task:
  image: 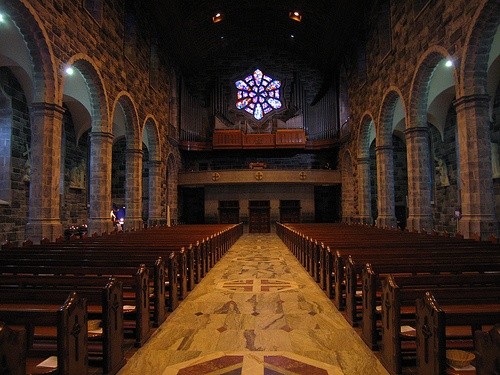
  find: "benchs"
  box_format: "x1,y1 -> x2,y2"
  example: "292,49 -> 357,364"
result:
0,220 -> 244,375
272,218 -> 499,375
